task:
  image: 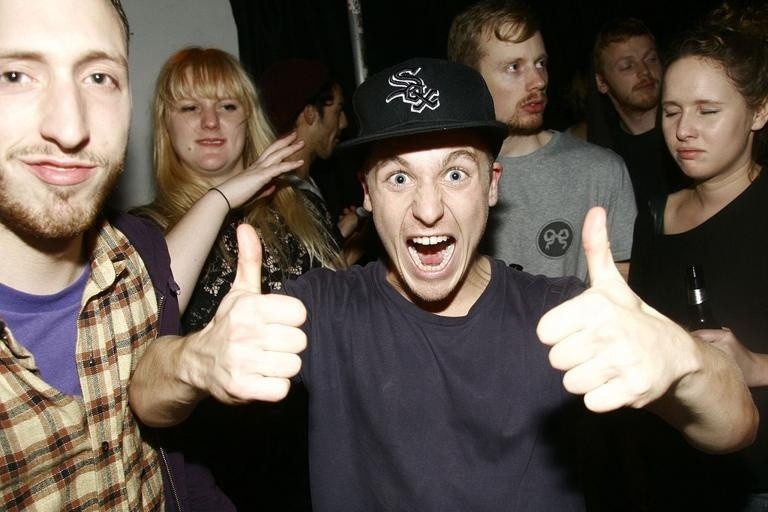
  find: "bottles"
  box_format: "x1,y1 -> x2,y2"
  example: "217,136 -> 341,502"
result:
680,266 -> 722,332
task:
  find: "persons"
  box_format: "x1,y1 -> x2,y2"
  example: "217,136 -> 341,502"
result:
126,45 -> 354,329
613,1 -> 768,510
0,0 -> 187,510
557,17 -> 692,193
255,44 -> 370,237
441,2 -> 642,297
126,46 -> 762,509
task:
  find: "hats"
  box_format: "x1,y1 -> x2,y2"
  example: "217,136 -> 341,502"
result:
334,54 -> 511,155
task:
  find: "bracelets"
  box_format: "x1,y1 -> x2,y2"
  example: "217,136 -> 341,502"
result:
205,180 -> 235,213
